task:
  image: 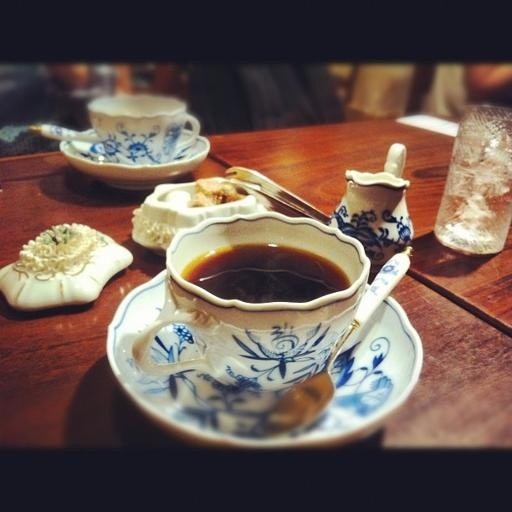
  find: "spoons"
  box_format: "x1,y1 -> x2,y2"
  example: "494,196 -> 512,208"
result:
262,247 -> 415,432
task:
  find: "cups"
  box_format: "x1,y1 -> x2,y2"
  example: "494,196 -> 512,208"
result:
88,96 -> 199,163
325,142 -> 415,279
139,216 -> 370,396
434,105 -> 511,255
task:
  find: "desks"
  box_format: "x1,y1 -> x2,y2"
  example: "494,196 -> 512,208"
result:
1,116 -> 512,449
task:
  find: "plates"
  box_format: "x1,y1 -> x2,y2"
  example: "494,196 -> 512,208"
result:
109,263 -> 424,447
60,130 -> 210,189
